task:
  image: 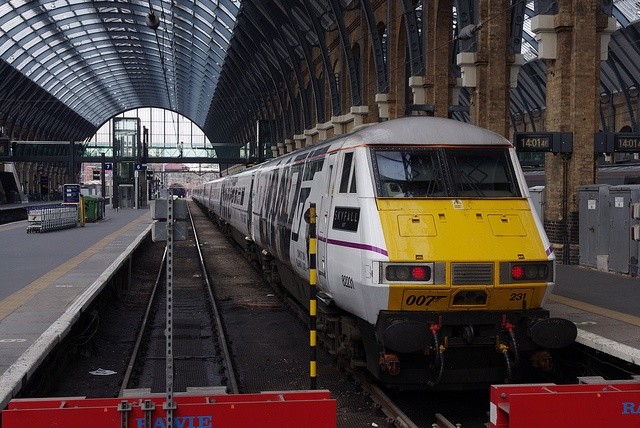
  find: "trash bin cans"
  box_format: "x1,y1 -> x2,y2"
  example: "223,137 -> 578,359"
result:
82,195 -> 98,223
96,196 -> 105,219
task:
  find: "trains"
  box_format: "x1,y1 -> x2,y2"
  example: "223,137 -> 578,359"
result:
191,116 -> 580,393
